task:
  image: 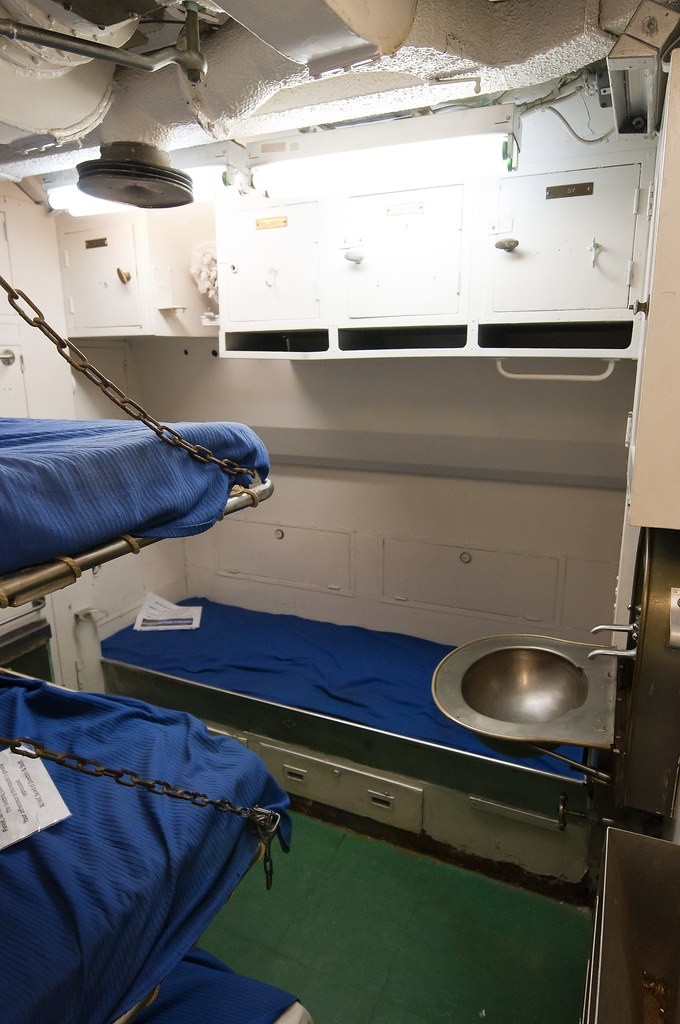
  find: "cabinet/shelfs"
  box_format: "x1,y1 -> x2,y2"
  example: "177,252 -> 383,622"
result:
214,145 -> 658,381
57,212 -> 217,339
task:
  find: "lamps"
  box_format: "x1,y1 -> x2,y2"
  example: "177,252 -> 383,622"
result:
42,139 -> 247,218
247,101 -> 522,203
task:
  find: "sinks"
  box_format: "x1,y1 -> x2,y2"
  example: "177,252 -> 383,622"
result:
431,633 -> 618,759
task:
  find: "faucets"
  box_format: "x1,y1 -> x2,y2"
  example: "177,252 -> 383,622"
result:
588,646 -> 637,661
590,616 -> 640,635
